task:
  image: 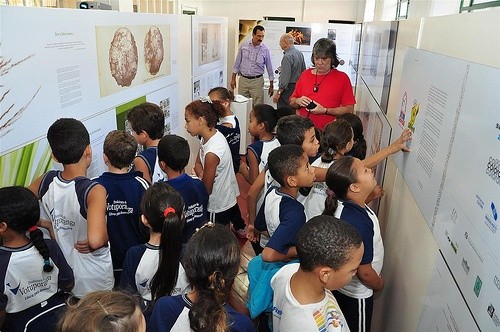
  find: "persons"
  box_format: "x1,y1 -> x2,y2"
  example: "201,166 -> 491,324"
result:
288,38 -> 356,131
273,34 -> 306,118
230,25 -> 274,108
0,87 -> 413,332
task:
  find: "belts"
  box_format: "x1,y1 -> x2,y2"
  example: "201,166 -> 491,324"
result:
241,74 -> 263,80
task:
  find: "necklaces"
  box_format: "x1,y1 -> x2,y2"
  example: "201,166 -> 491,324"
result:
313,69 -> 331,92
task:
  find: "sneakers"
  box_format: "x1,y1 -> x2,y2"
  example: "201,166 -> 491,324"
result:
232,224 -> 246,238
